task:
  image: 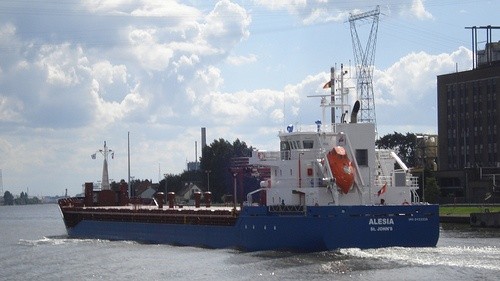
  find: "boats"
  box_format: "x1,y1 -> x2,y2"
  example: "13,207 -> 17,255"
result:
55,60 -> 441,253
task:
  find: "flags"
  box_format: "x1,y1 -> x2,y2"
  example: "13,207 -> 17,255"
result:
322,80 -> 332,89
378,184 -> 386,197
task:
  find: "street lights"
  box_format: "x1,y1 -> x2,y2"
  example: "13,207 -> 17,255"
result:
130,176 -> 135,197
232,171 -> 238,212
163,174 -> 168,204
205,169 -> 212,191
